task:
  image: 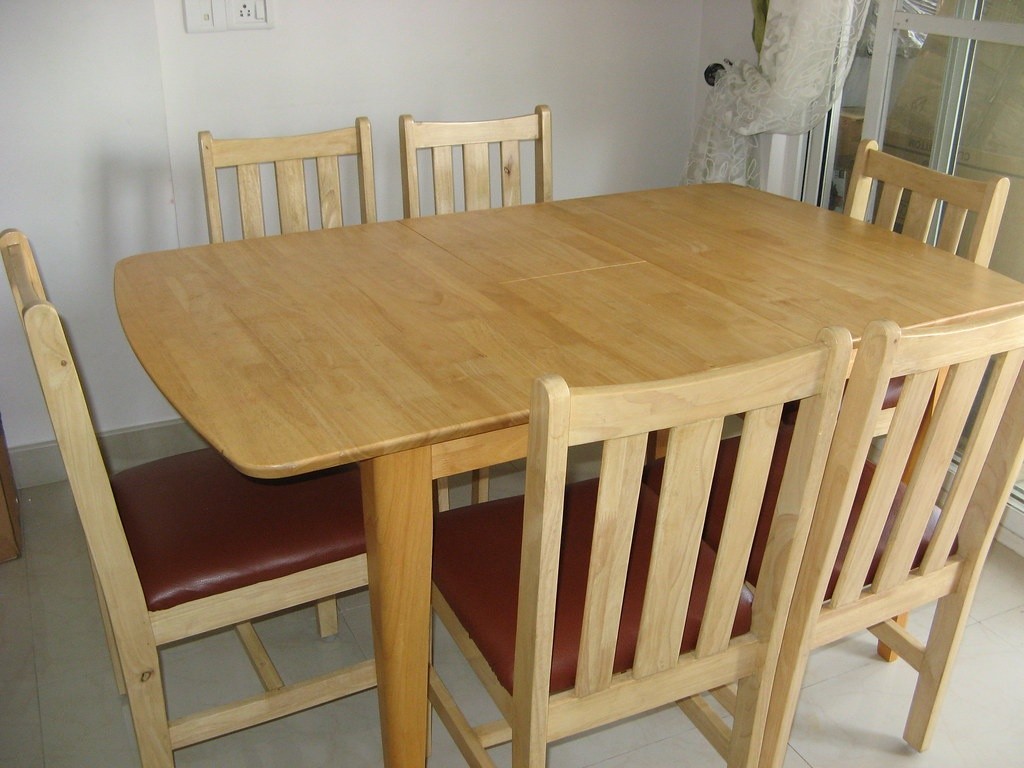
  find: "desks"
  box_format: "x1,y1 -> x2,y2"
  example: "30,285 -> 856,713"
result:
114,179 -> 1024,768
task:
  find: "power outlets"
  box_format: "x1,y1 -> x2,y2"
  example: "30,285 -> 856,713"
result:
225,0 -> 273,30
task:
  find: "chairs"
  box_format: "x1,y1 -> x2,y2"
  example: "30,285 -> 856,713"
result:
735,138 -> 1013,438
399,106 -> 554,505
0,229 -> 379,768
427,323 -> 854,768
198,117 -> 449,512
642,303 -> 1024,768
